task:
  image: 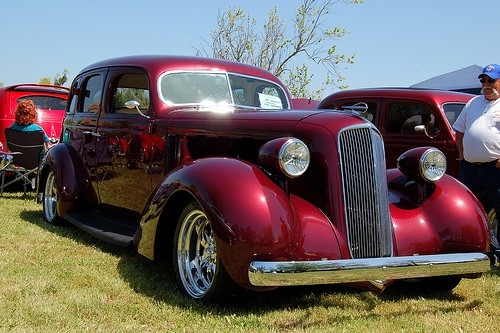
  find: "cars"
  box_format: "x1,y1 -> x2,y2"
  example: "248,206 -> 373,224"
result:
33,54 -> 500,308
0,84 -> 71,168
289,87 -> 479,181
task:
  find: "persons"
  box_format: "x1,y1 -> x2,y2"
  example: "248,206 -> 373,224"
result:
452,64 -> 500,261
10,98 -> 55,155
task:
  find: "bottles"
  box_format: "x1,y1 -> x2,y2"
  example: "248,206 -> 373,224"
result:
51,134 -> 56,143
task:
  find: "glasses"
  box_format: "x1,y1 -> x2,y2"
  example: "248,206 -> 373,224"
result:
480,78 -> 495,83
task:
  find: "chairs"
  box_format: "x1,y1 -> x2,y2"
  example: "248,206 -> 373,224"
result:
0,128 -> 55,199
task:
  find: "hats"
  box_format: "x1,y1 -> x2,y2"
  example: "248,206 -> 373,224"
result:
477,64 -> 500,80
